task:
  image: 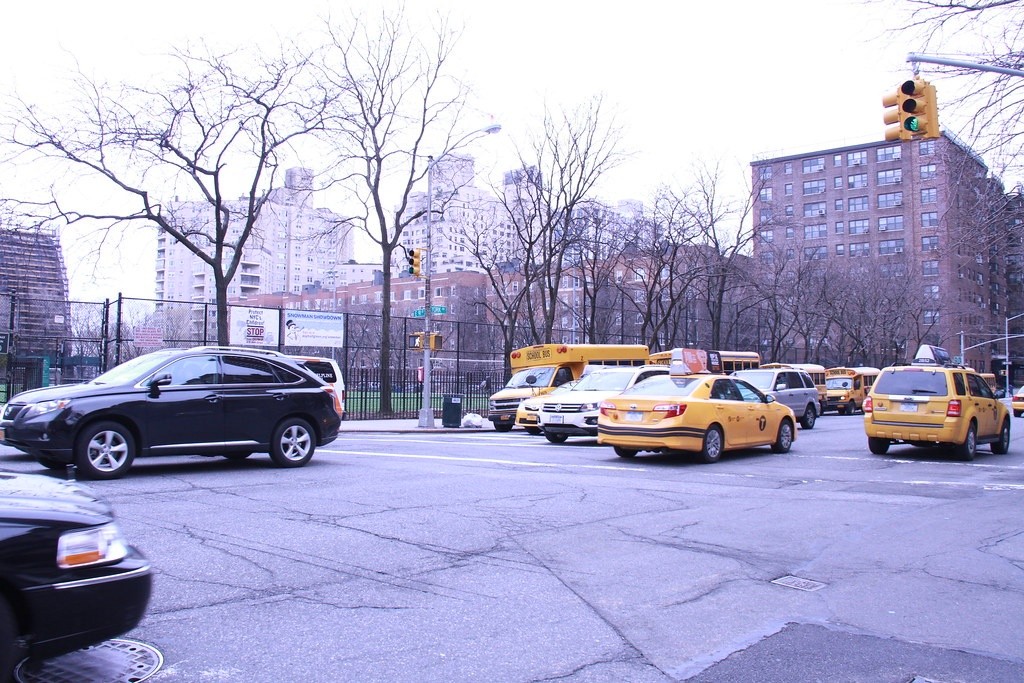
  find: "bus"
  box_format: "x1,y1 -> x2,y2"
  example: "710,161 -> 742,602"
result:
759,363 -> 829,415
978,373 -> 997,395
650,350 -> 760,377
825,366 -> 880,416
487,343 -> 651,432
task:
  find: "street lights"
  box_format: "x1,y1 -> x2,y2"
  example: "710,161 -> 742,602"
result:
418,123 -> 503,428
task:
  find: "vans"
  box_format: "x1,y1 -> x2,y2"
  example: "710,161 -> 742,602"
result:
287,356 -> 346,419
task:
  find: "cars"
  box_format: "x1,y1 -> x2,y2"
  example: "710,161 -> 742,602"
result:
596,347 -> 798,463
1011,385 -> 1024,417
1,471 -> 155,683
514,380 -> 580,435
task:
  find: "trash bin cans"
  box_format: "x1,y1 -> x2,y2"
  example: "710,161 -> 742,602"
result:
442,393 -> 464,428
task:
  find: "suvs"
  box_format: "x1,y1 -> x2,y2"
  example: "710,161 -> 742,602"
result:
862,343 -> 1010,461
728,367 -> 822,430
535,364 -> 714,444
0,346 -> 343,481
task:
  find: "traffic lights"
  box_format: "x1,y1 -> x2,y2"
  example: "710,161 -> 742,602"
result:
882,89 -> 902,143
429,334 -> 443,350
408,332 -> 424,350
901,77 -> 930,136
408,247 -> 421,275
